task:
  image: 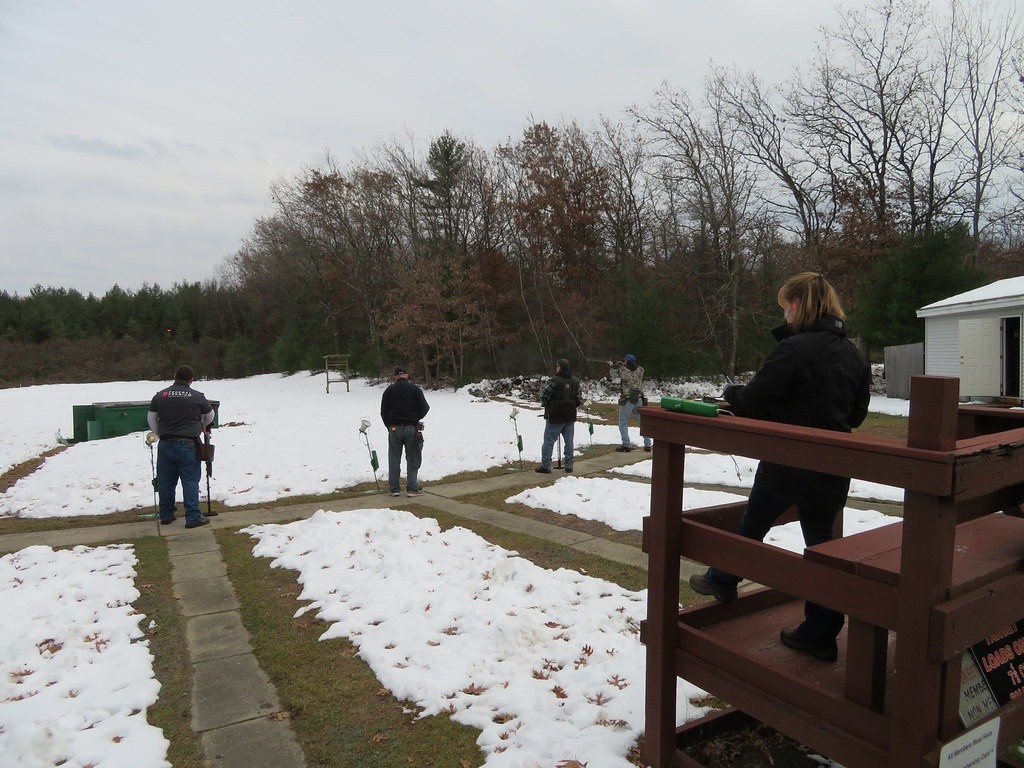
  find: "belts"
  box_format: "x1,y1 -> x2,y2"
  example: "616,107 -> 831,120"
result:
160,436 -> 196,440
393,423 -> 416,425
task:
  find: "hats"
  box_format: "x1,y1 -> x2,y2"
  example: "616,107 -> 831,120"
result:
623,354 -> 635,360
557,359 -> 569,368
394,367 -> 410,379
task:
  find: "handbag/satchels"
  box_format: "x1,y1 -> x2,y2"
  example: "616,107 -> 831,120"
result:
618,398 -> 626,406
549,400 -> 576,424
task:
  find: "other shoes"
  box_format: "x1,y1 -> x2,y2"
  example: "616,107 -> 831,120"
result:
780,629 -> 838,662
615,445 -> 630,452
407,490 -> 424,497
645,447 -> 651,451
185,517 -> 210,528
535,467 -> 551,473
690,574 -> 737,601
565,468 -> 573,473
388,490 -> 402,496
161,515 -> 177,525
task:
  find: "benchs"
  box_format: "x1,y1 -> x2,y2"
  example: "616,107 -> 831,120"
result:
804,513 -> 1024,715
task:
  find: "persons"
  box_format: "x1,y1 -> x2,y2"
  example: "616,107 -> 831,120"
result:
535,359 -> 583,474
689,272 -> 872,664
146,366 -> 215,530
607,354 -> 651,452
380,367 -> 430,497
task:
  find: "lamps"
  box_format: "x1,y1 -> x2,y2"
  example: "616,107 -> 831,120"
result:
508,408 -> 526,472
358,416 -> 384,494
145,432 -> 161,535
583,399 -> 596,452
